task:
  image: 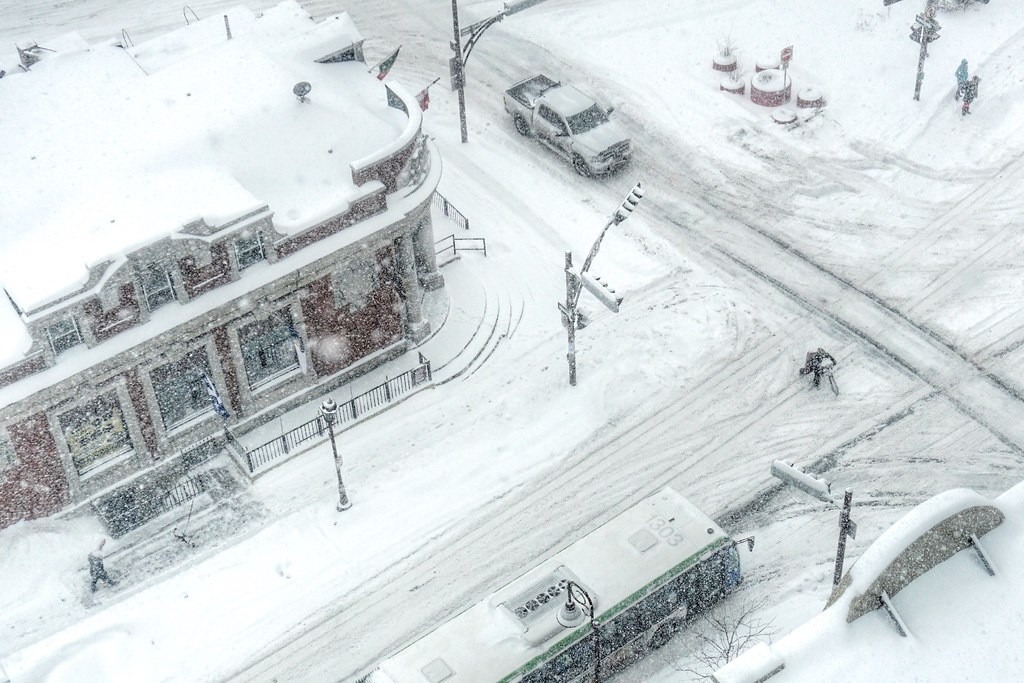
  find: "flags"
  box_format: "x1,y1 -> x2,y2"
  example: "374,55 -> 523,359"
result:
377,49 -> 399,81
415,82 -> 433,111
201,372 -> 231,420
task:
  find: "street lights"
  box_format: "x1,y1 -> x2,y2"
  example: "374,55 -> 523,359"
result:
451,1 -> 545,144
560,580 -> 604,683
320,396 -> 353,511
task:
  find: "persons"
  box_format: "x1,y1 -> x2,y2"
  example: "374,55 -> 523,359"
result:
800,347 -> 836,387
87,539 -> 121,592
954,59 -> 981,115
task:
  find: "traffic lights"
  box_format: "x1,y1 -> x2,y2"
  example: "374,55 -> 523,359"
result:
613,181 -> 646,221
581,269 -> 624,313
771,459 -> 833,504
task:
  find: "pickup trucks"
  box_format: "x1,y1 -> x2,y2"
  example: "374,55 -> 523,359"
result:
504,72 -> 633,179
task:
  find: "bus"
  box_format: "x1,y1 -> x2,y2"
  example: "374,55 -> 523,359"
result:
356,483 -> 758,683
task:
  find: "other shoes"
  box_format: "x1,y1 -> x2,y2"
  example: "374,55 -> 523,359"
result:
966,109 -> 971,114
962,109 -> 965,115
